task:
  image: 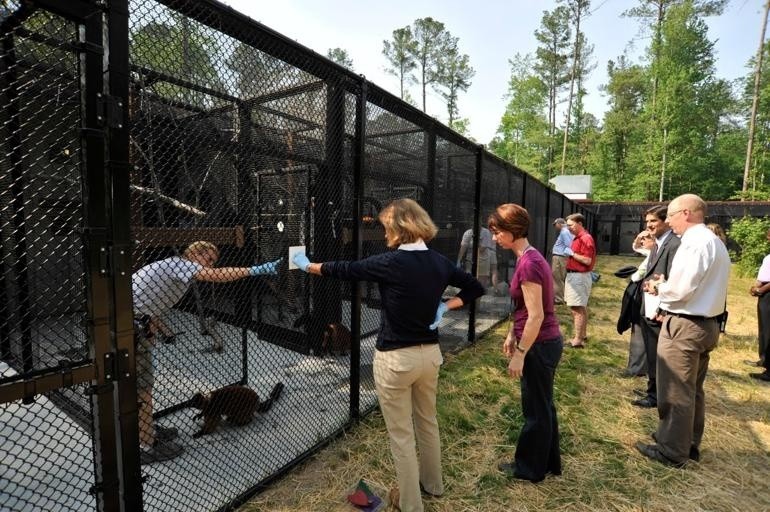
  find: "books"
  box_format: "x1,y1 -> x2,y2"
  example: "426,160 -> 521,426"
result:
643,291 -> 662,319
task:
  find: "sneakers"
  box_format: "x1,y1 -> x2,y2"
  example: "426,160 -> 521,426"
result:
390,487 -> 401,510
139,424 -> 184,464
499,461 -> 515,476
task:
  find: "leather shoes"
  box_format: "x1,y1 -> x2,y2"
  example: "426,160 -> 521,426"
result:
743,360 -> 758,367
631,397 -> 656,408
750,373 -> 770,381
633,387 -> 649,397
635,440 -> 671,467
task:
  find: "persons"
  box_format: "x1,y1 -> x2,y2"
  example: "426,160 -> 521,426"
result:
563,211 -> 596,348
294,198 -> 494,511
131,238 -> 283,464
550,217 -> 573,305
489,203 -> 565,485
742,227 -> 770,383
613,205 -> 731,409
457,210 -> 498,315
636,192 -> 731,473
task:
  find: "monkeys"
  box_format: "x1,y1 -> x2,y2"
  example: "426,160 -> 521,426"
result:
190,382 -> 285,439
321,321 -> 351,358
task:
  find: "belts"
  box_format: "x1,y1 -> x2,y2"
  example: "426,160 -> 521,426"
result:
566,269 -> 577,273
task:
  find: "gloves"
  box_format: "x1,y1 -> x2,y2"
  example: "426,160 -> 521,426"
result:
251,257 -> 283,278
430,303 -> 449,328
292,250 -> 309,272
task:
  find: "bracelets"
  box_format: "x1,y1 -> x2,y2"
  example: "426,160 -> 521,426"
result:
654,281 -> 662,293
571,252 -> 574,257
756,288 -> 760,294
516,344 -> 528,356
304,264 -> 313,273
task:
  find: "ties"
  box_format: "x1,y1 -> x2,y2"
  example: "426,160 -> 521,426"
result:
647,241 -> 658,271
